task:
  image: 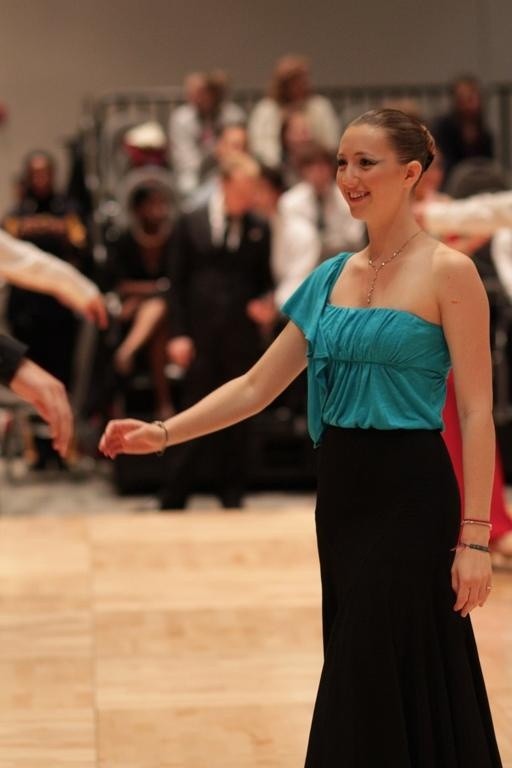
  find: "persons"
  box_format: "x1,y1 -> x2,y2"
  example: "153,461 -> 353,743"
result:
99,108 -> 503,768
0,52 -> 512,558
